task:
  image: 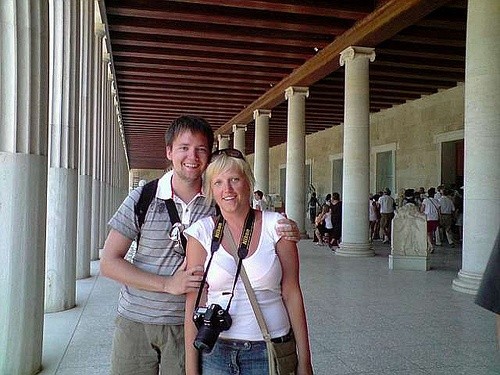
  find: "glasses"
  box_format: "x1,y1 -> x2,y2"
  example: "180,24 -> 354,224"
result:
209,148 -> 246,162
168,225 -> 185,256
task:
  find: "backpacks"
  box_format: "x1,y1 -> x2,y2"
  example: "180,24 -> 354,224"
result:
405,197 -> 416,205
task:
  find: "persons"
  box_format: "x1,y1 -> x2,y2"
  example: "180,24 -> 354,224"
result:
100,116 -> 301,375
181,148 -> 316,375
253,182 -> 466,253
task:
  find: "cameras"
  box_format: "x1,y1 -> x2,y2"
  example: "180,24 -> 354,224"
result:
191,304 -> 232,354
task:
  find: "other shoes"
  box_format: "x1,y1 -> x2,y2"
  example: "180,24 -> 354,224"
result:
430,248 -> 434,253
382,239 -> 389,243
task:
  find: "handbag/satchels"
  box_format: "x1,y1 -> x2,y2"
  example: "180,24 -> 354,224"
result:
266,335 -> 299,375
